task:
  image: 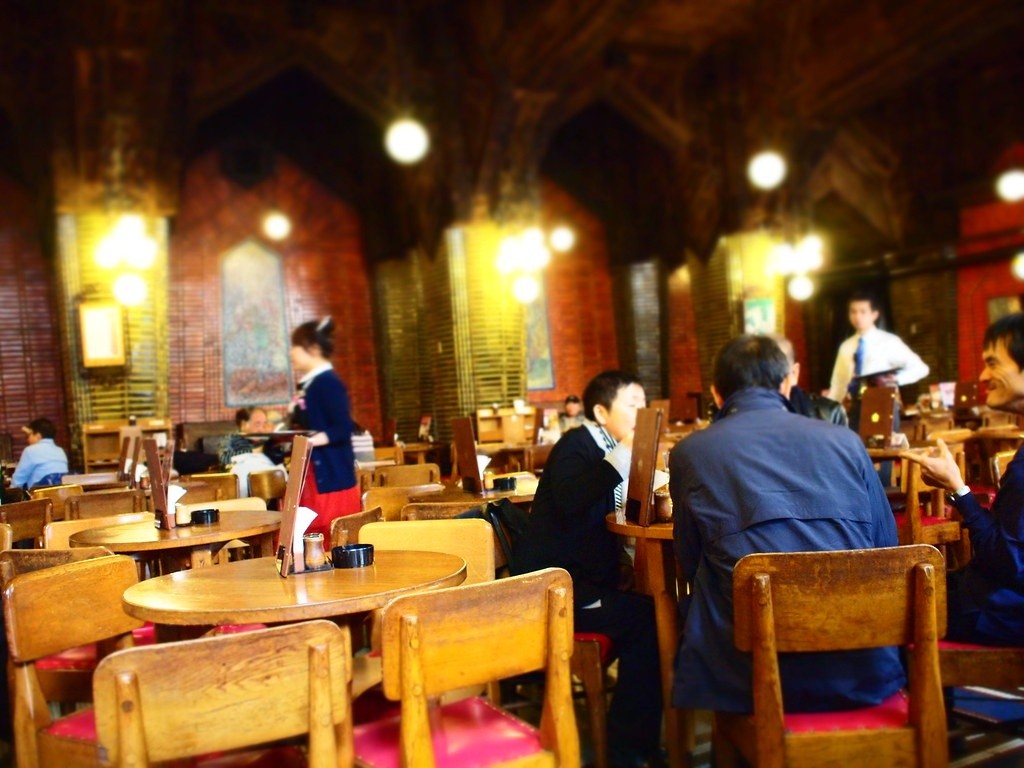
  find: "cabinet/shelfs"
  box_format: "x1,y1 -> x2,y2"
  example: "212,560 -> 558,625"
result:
81,415 -> 173,474
477,409 -> 536,450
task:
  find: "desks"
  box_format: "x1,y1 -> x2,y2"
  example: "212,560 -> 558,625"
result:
69,510 -> 281,576
409,488 -> 536,512
403,441 -> 443,465
606,510 -> 695,768
123,550 -> 467,656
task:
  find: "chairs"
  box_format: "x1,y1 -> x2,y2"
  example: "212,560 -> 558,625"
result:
0,411 -> 1023,768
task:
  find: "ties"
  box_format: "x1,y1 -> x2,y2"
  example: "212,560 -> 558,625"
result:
848,339 -> 867,399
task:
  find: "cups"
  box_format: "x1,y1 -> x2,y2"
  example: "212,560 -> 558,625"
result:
653,492 -> 671,523
484,472 -> 494,490
302,533 -> 326,570
140,476 -> 150,489
175,505 -> 191,524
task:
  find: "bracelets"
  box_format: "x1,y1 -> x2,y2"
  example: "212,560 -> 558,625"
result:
945,486 -> 970,504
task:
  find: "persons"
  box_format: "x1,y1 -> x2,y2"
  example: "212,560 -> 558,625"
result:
558,297 -> 930,433
0,406 -> 286,492
667,334 -> 910,713
897,313 -> 1024,647
508,370 -> 673,768
252,315 -> 362,557
